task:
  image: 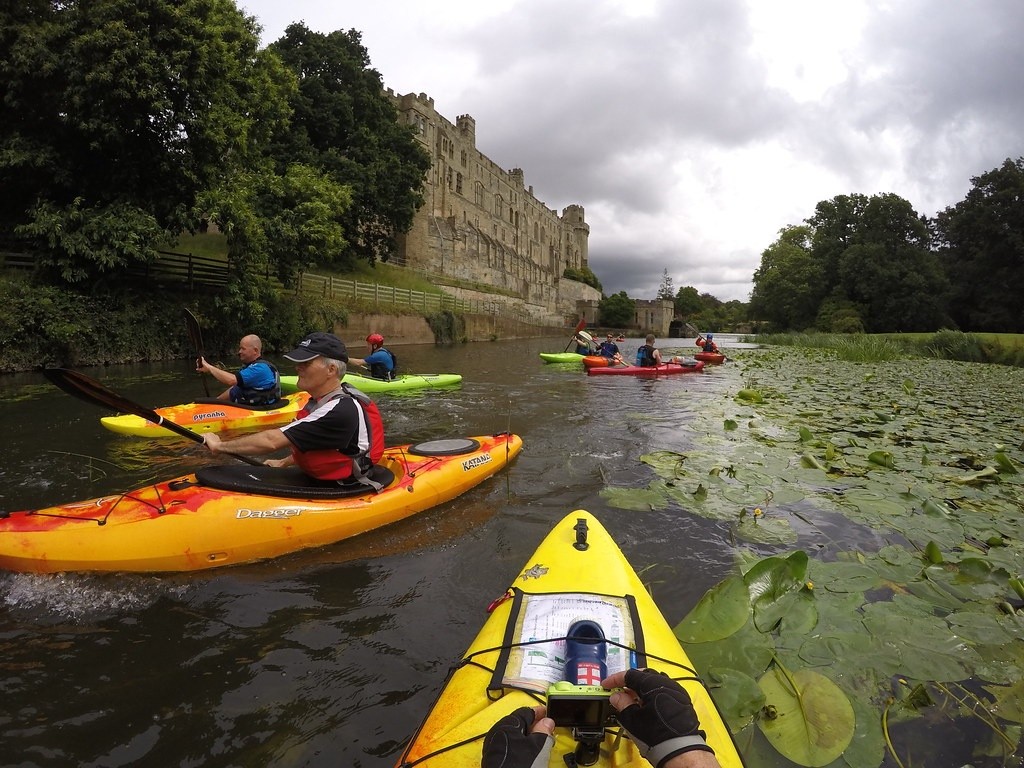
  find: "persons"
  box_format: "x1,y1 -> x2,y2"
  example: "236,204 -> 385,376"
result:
347,333 -> 397,379
200,332 -> 384,480
481,668 -> 722,768
596,331 -> 620,360
196,334 -> 281,406
636,334 -> 662,367
571,334 -> 590,354
696,334 -> 718,352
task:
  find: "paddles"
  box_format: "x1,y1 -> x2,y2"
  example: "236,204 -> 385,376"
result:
578,330 -> 628,367
183,308 -> 211,396
41,365 -> 265,468
661,361 -> 698,366
564,319 -> 586,352
685,322 -> 732,361
360,365 -> 371,373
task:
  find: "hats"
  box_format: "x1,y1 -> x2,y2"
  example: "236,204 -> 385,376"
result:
282,332 -> 348,363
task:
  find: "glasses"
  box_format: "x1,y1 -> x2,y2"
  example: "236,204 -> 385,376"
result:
607,336 -> 613,338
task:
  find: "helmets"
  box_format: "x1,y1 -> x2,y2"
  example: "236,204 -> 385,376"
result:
707,334 -> 713,339
366,333 -> 384,346
